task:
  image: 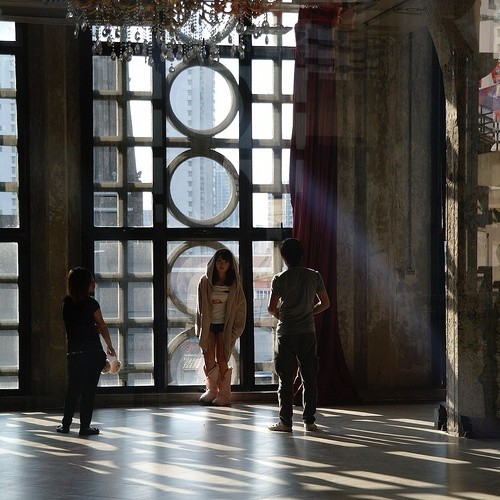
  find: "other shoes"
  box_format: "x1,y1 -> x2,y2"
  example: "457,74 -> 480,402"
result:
56,425 -> 70,433
79,427 -> 100,436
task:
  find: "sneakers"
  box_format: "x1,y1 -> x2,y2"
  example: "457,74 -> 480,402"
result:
304,422 -> 318,431
267,422 -> 293,432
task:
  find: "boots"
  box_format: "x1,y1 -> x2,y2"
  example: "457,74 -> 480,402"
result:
199,363 -> 219,406
212,366 -> 233,406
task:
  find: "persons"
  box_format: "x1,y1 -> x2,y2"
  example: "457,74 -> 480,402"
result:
56,267 -> 115,436
195,249 -> 247,405
267,239 -> 331,432
